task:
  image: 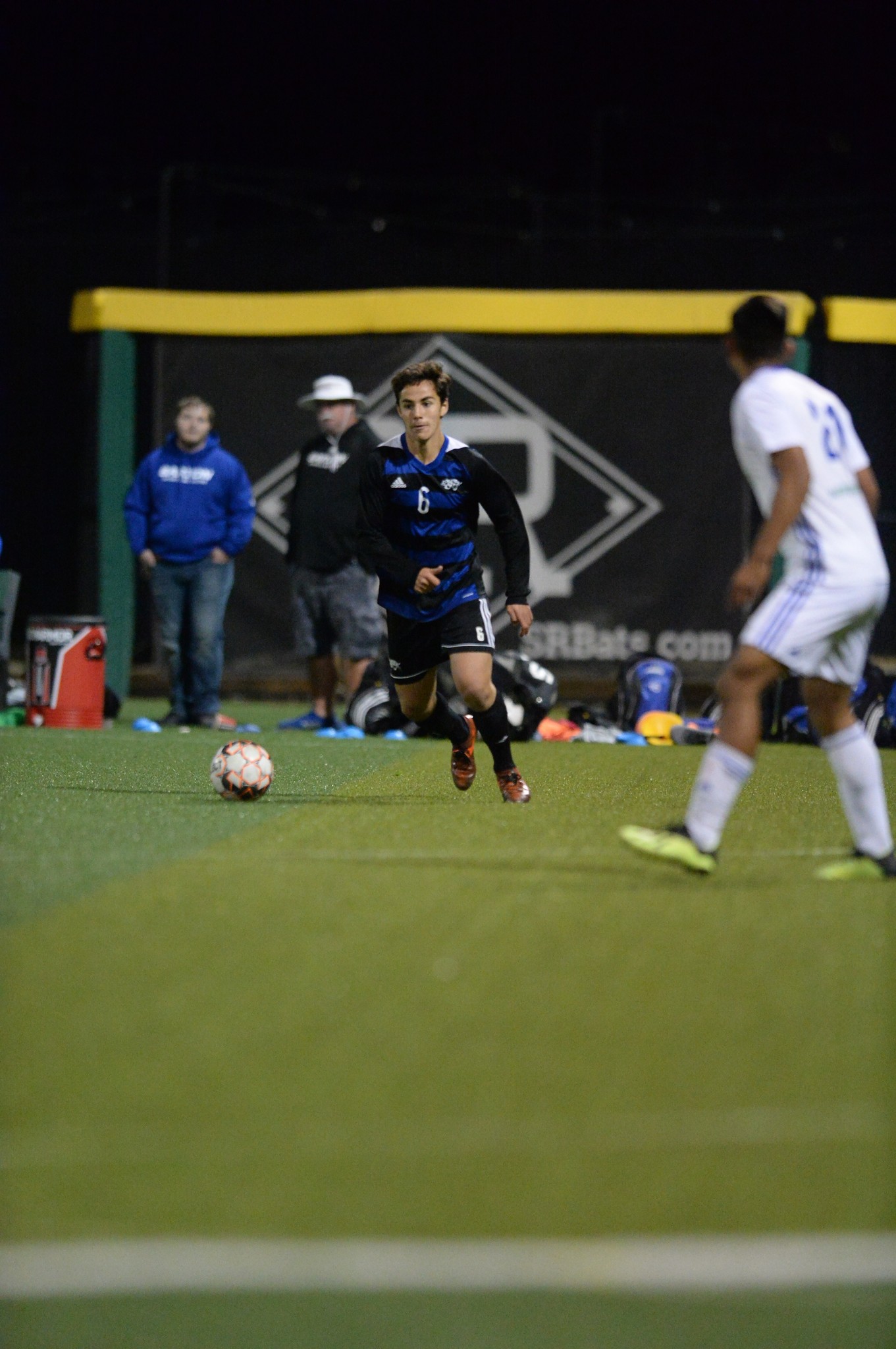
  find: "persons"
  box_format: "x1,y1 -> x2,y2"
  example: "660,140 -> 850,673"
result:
273,369 -> 384,737
596,286 -> 896,883
117,390 -> 252,735
343,356 -> 542,802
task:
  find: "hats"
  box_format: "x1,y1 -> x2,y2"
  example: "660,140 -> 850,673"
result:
296,376 -> 368,414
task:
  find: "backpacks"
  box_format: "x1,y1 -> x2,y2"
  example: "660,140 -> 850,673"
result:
340,623 -> 896,751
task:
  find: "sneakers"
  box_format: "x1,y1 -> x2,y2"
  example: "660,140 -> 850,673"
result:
816,853 -> 896,882
451,716 -> 478,791
154,711 -> 193,727
282,711 -> 337,734
493,763 -> 530,804
192,714 -> 236,729
618,820 -> 715,877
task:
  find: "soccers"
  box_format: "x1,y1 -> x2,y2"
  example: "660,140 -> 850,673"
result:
210,741 -> 273,801
